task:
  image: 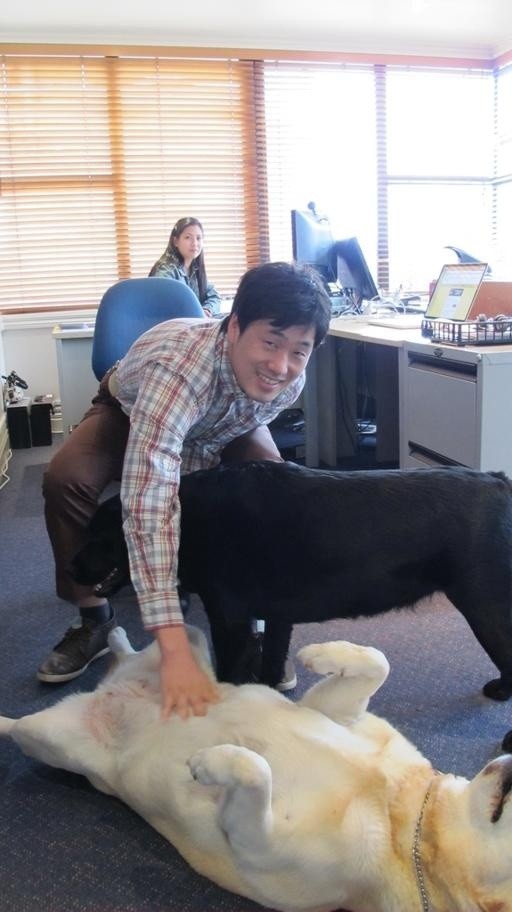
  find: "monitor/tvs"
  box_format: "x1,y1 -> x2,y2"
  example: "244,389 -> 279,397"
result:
291,209 -> 338,297
335,237 -> 381,311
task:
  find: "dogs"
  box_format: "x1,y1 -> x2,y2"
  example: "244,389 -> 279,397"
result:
71,456 -> 511,756
0,625 -> 512,912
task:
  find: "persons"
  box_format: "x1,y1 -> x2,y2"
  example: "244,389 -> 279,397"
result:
38,261 -> 331,686
146,216 -> 221,320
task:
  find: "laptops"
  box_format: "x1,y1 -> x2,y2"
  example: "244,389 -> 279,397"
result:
366,263 -> 488,330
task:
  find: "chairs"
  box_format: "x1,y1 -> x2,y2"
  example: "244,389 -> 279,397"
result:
91,277 -> 204,619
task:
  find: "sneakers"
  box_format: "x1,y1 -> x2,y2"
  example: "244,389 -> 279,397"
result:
35,606 -> 119,683
274,657 -> 299,692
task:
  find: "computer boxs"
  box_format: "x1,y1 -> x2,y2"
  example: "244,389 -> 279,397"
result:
7,397 -> 31,449
30,394 -> 52,446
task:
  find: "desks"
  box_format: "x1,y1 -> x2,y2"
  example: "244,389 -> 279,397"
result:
304,313 -> 512,480
